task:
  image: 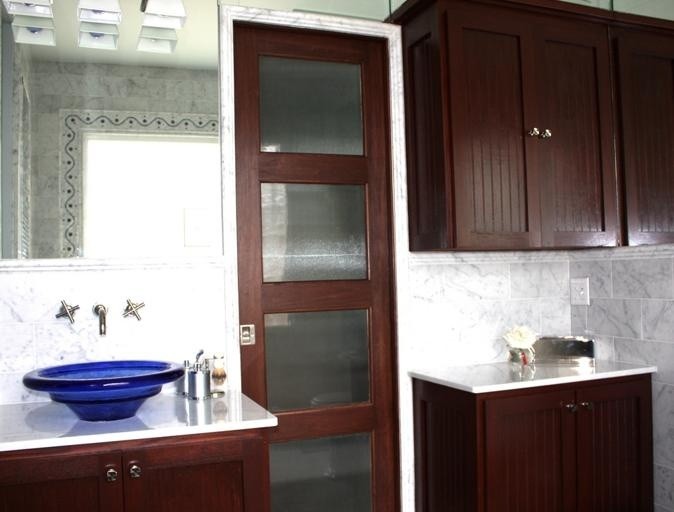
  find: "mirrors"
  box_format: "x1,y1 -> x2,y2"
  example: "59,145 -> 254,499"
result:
0,1 -> 220,260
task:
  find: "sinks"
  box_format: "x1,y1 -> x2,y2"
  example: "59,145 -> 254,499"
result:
22,359 -> 187,423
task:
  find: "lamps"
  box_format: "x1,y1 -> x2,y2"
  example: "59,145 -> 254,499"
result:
5,1 -> 186,54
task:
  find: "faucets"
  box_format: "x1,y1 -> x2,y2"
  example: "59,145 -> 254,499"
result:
93,302 -> 110,337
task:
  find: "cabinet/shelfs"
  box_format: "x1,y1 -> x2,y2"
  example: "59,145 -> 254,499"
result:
388,1 -> 622,252
411,373 -> 659,512
611,13 -> 674,248
0,428 -> 280,512
